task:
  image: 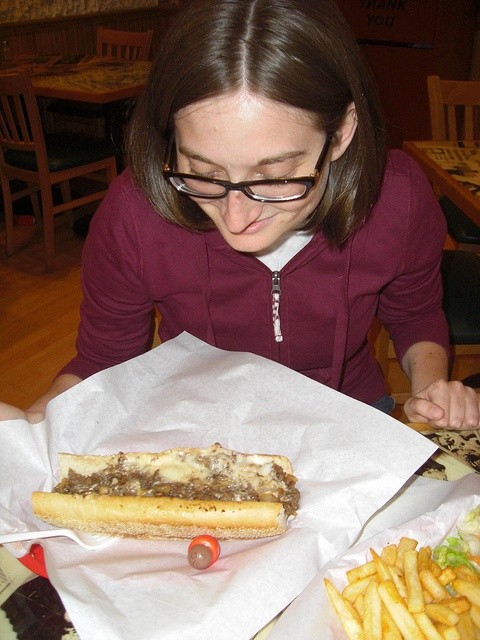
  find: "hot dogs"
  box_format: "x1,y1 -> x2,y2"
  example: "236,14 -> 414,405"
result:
31,443 -> 301,541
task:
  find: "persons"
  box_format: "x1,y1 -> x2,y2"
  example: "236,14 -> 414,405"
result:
2,2 -> 479,437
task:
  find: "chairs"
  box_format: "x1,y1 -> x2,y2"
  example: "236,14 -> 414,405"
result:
424,69 -> 479,256
0,72 -> 122,259
362,248 -> 479,407
87,26 -> 155,64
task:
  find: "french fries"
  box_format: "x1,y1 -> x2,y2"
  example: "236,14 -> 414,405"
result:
324,537 -> 479,640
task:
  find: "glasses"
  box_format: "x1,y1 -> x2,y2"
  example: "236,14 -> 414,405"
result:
164,136 -> 327,203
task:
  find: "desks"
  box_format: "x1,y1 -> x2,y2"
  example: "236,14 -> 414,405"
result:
1,51 -> 152,112
397,133 -> 480,226
